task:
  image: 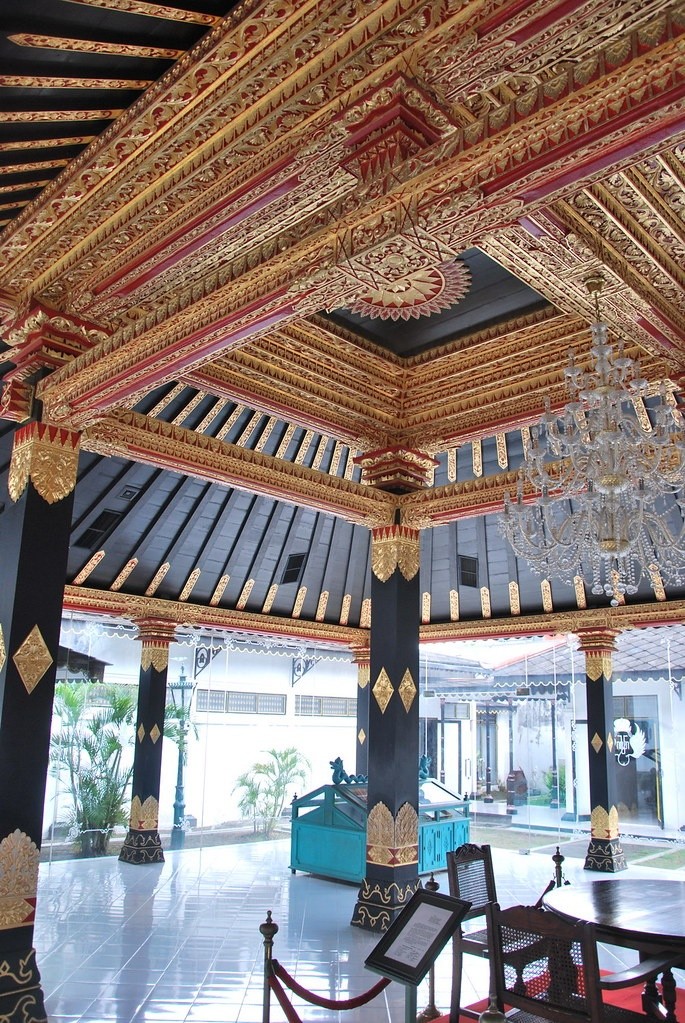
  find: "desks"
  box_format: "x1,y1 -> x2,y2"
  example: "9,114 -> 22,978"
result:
542,878 -> 685,1023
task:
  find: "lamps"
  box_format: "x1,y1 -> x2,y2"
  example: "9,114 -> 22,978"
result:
422,644 -> 436,697
497,275 -> 685,608
516,638 -> 530,696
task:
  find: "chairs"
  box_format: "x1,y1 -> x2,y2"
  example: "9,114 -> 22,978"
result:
447,844 -> 681,1023
